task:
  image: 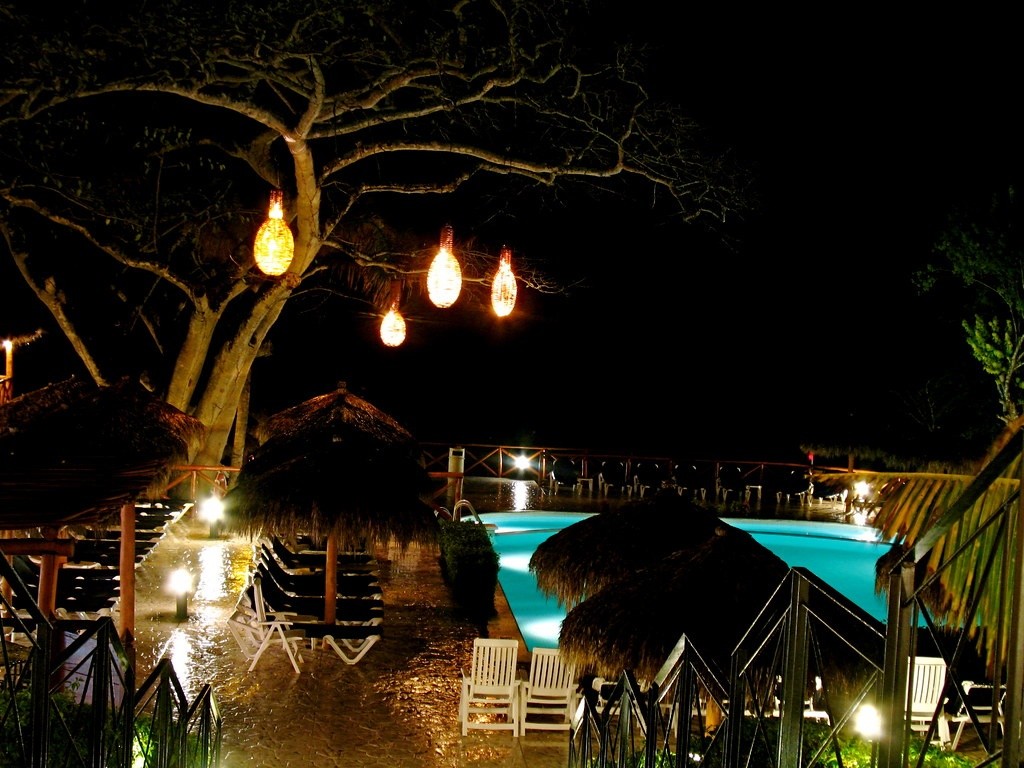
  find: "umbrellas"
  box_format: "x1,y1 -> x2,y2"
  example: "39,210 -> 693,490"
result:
232,382 -> 443,623
528,485 -> 887,768
183,205 -> 497,311
0,374 -> 207,632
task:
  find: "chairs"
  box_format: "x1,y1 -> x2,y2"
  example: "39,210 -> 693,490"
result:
0,498 -> 195,643
904,656 -> 1006,751
460,637 -> 522,737
227,531 -> 385,673
547,458 -> 879,515
572,675 -> 831,738
520,647 -> 577,736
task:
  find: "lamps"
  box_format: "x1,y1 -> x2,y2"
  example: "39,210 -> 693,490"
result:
427,223 -> 463,306
254,162 -> 295,276
491,245 -> 517,314
379,279 -> 406,347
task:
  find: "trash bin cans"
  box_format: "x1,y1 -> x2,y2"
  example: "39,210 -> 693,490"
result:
448,447 -> 465,482
